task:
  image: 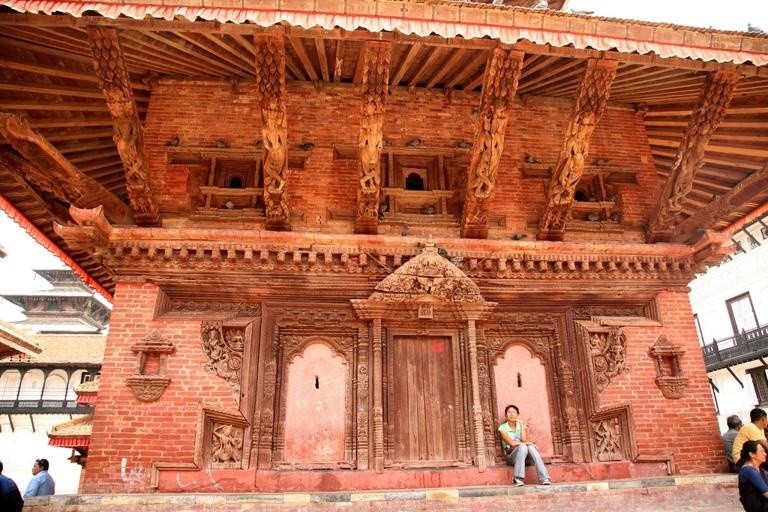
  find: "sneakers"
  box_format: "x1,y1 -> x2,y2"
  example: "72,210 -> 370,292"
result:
538,479 -> 551,485
512,477 -> 525,486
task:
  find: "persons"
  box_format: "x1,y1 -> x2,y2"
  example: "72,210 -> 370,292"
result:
497,404 -> 551,486
596,423 -> 615,455
735,440 -> 768,512
23,458 -> 56,498
0,459 -> 24,512
213,425 -> 239,463
731,408 -> 768,471
722,415 -> 744,466
